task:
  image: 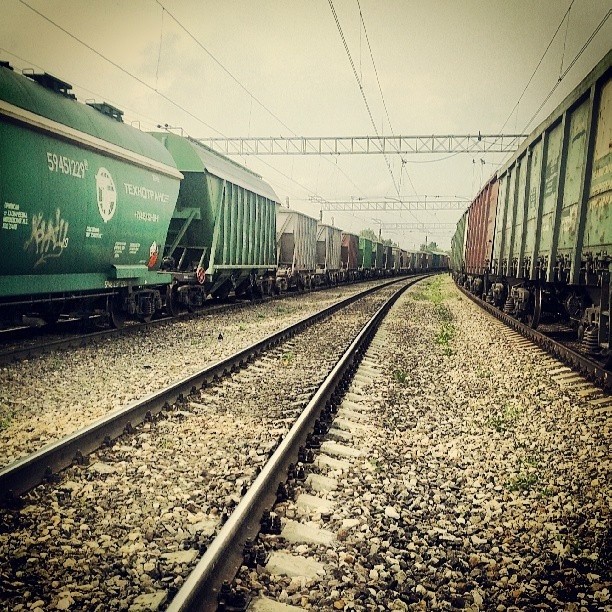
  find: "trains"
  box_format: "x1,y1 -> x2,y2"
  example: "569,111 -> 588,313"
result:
0,63 -> 449,328
451,49 -> 611,364
